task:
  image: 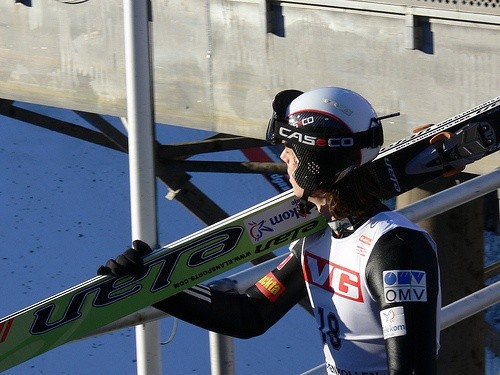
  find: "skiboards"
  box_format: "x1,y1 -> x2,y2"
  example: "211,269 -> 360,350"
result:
0,95 -> 500,374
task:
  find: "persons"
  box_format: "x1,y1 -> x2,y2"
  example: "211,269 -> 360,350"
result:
99,86 -> 442,375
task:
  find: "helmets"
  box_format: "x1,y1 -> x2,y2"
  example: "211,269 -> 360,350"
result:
264,87 -> 384,194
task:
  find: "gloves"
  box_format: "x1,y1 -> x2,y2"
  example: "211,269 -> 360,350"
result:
98,239 -> 154,277
